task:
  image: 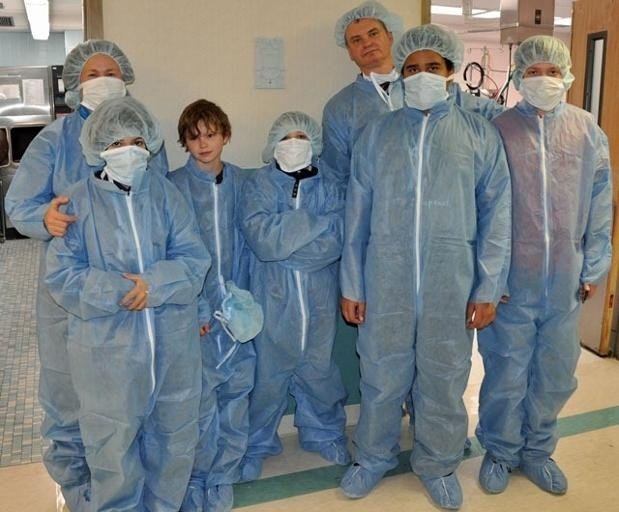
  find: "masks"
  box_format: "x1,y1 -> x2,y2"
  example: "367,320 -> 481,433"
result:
79,77 -> 126,110
520,76 -> 565,111
403,72 -> 449,111
100,145 -> 150,186
365,68 -> 399,84
274,138 -> 312,173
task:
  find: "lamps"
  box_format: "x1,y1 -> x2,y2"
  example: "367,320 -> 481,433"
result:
22,0 -> 51,41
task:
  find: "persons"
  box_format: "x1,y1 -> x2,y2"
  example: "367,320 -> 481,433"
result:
3,37 -> 169,512
163,97 -> 259,512
44,98 -> 212,512
338,22 -> 511,510
323,1 -> 504,455
240,109 -> 352,483
472,34 -> 613,499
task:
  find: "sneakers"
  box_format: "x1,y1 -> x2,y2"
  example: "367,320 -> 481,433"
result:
479,458 -> 567,495
185,459 -> 261,512
423,473 -> 463,510
319,441 -> 382,498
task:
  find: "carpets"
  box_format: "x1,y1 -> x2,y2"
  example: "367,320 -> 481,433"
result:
0,215 -> 54,469
